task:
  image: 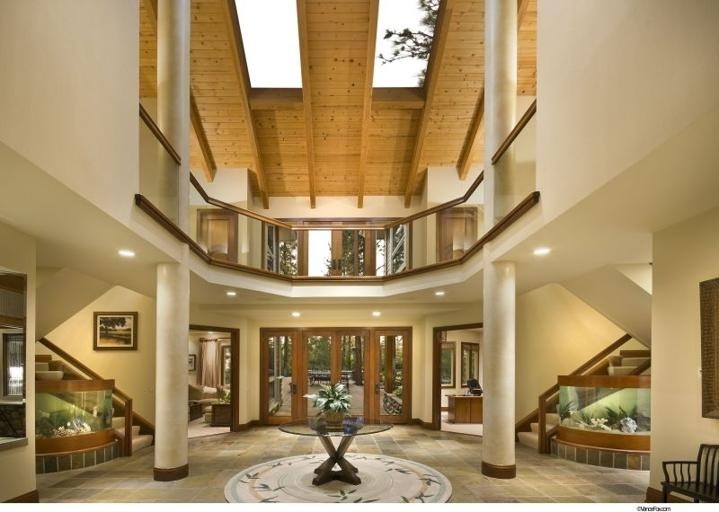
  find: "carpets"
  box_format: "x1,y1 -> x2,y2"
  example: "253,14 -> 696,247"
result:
222,454 -> 454,503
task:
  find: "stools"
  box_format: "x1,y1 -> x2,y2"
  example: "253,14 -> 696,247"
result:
204,405 -> 212,423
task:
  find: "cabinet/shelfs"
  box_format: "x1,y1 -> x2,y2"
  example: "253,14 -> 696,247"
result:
447,394 -> 483,423
210,401 -> 232,426
190,403 -> 203,421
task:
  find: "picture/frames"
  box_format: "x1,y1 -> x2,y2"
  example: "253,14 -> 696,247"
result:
188,353 -> 196,372
92,310 -> 139,352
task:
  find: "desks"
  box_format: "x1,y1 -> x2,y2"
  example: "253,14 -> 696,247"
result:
277,421 -> 394,486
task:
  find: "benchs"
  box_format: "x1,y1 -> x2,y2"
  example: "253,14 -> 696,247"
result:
660,443 -> 719,503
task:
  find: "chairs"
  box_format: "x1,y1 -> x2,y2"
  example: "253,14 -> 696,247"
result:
310,367 -> 349,394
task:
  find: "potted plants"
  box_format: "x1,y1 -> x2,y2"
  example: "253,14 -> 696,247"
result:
300,382 -> 353,426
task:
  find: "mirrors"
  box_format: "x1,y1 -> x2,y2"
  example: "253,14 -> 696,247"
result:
1,272 -> 27,441
